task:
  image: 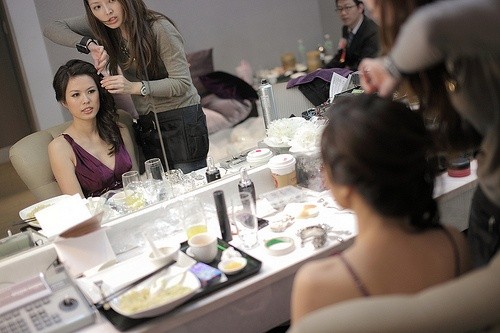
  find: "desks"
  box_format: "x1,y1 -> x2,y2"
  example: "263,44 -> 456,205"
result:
73,160 -> 479,333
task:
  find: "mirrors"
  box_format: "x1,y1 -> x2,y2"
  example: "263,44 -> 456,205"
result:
0,0 -> 381,260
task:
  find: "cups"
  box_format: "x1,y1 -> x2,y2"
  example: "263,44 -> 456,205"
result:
144,157 -> 165,181
121,170 -> 141,193
178,198 -> 207,238
247,148 -> 274,168
187,231 -> 218,264
267,153 -> 299,187
230,191 -> 259,250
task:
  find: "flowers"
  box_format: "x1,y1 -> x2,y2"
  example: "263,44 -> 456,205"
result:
338,36 -> 347,48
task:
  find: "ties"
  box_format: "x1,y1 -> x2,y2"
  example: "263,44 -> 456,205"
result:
347,31 -> 354,50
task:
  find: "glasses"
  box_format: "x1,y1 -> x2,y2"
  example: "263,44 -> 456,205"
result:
335,3 -> 359,14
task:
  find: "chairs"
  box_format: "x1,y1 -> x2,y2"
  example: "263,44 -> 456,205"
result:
9,109 -> 147,202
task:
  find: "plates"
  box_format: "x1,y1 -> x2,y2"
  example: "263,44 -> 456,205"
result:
18,194 -> 72,229
217,257 -> 247,275
101,263 -> 201,320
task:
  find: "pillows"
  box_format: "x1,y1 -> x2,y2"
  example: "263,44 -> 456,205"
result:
197,71 -> 259,102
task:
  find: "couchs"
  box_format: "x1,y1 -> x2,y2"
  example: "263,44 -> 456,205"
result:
186,47 -> 252,134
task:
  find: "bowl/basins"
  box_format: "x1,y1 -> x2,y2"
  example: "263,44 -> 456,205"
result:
147,241 -> 182,267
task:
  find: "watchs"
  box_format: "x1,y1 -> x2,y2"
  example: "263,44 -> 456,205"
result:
139,82 -> 147,97
76,36 -> 94,54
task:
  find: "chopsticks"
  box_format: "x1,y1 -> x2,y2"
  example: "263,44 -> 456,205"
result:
92,259 -> 178,308
11,216 -> 37,227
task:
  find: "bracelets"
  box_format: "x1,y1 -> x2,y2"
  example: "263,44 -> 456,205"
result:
87,41 -> 97,46
380,56 -> 402,79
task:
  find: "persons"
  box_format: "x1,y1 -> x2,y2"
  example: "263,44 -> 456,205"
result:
325,0 -> 381,71
285,0 -> 500,333
49,59 -> 140,199
43,0 -> 209,174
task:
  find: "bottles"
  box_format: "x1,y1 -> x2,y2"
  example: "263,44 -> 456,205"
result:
259,78 -> 277,128
237,169 -> 256,216
206,158 -> 220,182
297,40 -> 307,65
324,34 -> 335,57
